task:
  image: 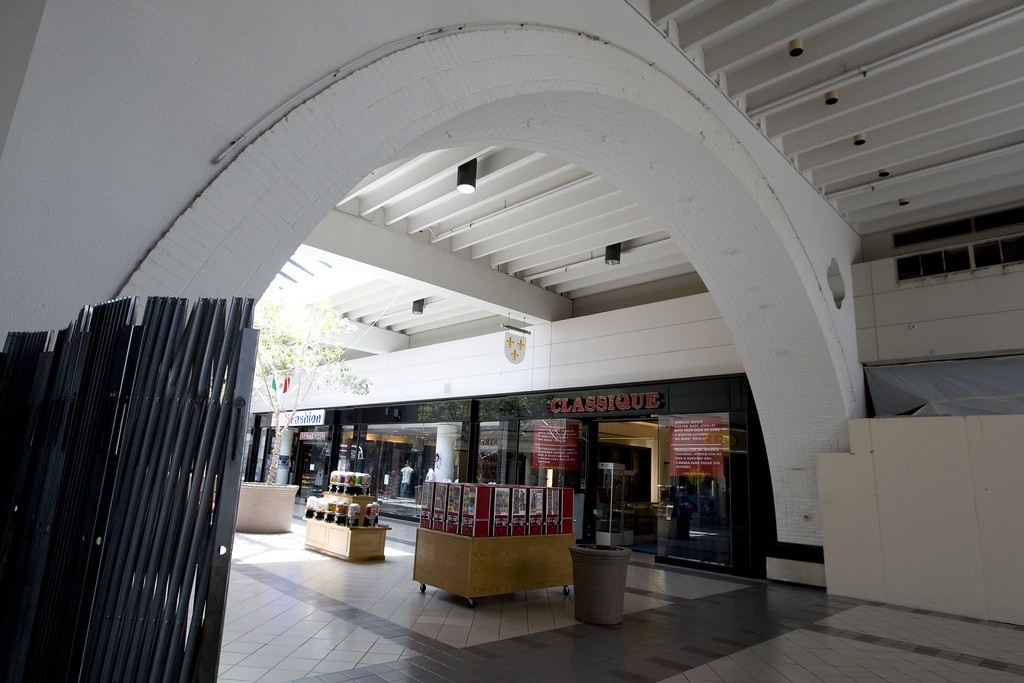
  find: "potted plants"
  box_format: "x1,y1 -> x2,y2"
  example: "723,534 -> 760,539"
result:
219,261 -> 378,536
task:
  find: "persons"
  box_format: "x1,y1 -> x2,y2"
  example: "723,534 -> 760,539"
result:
399,460 -> 413,497
424,467 -> 434,481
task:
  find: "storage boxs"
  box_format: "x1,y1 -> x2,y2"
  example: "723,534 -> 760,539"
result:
421,481 -> 575,538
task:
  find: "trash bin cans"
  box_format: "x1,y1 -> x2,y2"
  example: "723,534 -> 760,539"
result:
568,543 -> 633,625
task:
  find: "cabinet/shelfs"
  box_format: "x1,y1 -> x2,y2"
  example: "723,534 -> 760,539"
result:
595,463 -> 626,548
301,491 -> 392,563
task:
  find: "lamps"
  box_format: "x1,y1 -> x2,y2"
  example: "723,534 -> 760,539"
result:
788,36 -> 804,58
899,197 -> 910,206
878,168 -> 890,178
854,133 -> 866,146
604,242 -> 622,266
412,299 -> 424,315
824,90 -> 839,106
457,156 -> 477,194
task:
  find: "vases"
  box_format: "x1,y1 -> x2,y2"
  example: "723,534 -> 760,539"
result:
568,544 -> 632,626
